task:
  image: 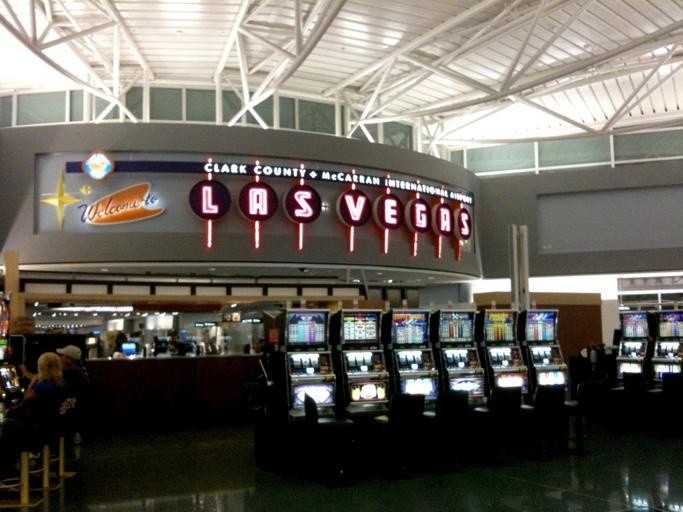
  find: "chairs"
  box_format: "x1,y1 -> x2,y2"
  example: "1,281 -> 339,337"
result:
250,387 -> 582,480
0,372 -> 94,507
623,373 -> 681,436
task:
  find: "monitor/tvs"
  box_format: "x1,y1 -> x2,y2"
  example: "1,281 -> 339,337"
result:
652,342 -> 683,362
0,365 -> 20,393
153,339 -> 168,357
121,342 -> 137,358
286,345 -> 568,377
617,341 -> 647,361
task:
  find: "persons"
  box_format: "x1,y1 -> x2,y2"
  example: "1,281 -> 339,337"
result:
85,321 -> 222,357
0,350 -> 63,472
17,345 -> 94,457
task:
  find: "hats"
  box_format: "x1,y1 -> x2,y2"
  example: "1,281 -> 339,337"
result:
56,345 -> 81,358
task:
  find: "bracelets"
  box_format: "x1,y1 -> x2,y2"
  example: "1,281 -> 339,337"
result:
27,384 -> 32,388
